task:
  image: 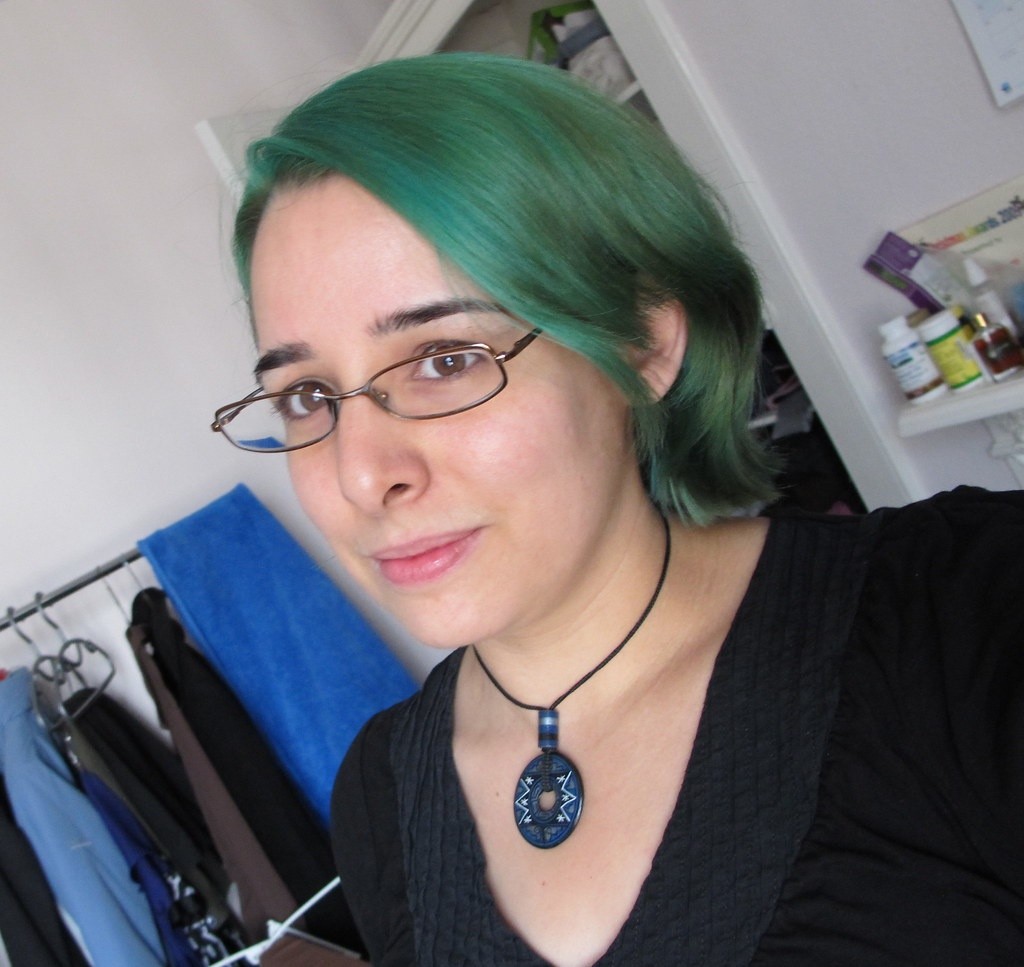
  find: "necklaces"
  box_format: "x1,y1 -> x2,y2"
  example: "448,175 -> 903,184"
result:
472,495 -> 672,849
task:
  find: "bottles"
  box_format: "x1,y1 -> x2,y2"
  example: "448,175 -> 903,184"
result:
962,258 -> 1022,349
919,311 -> 984,396
877,315 -> 950,404
970,311 -> 1023,381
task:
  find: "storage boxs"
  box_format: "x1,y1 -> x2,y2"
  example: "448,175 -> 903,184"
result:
863,232 -> 960,310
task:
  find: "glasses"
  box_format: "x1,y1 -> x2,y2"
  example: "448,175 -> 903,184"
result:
210,327 -> 542,452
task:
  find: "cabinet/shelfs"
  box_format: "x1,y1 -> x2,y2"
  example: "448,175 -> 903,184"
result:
187,0 -> 913,515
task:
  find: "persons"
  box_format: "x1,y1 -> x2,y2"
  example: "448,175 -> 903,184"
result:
229,53 -> 1024,967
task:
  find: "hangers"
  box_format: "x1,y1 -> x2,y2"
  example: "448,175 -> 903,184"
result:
96,565 -> 134,627
34,592 -> 117,728
7,607 -> 92,734
120,550 -> 157,611
